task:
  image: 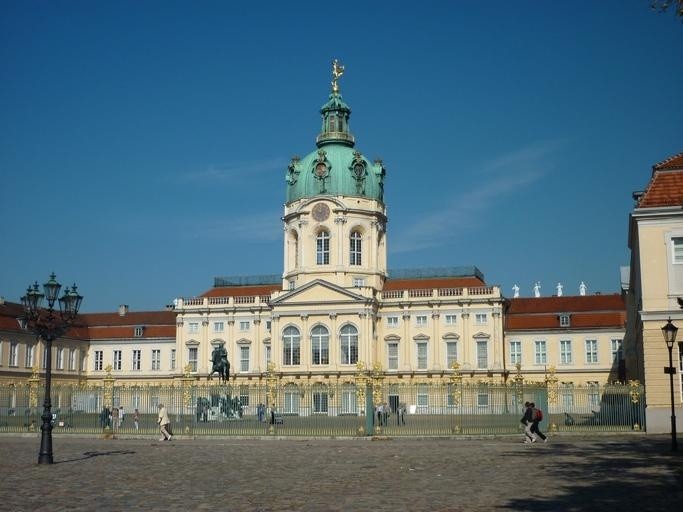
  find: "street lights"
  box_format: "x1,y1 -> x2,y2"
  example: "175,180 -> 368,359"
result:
18,272 -> 84,466
661,316 -> 681,445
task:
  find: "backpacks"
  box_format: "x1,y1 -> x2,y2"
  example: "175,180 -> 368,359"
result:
533,409 -> 543,422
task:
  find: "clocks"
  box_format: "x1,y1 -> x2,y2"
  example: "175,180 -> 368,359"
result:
311,202 -> 330,222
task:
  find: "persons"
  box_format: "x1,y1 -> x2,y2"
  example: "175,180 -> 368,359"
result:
530,281 -> 544,298
155,403 -> 173,442
373,401 -> 408,426
578,282 -> 589,296
132,408 -> 142,432
520,401 -> 550,444
554,281 -> 564,297
258,403 -> 276,424
99,406 -> 126,432
511,283 -> 520,298
216,343 -> 230,379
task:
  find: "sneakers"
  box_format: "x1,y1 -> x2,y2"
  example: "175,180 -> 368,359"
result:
523,436 -> 548,444
